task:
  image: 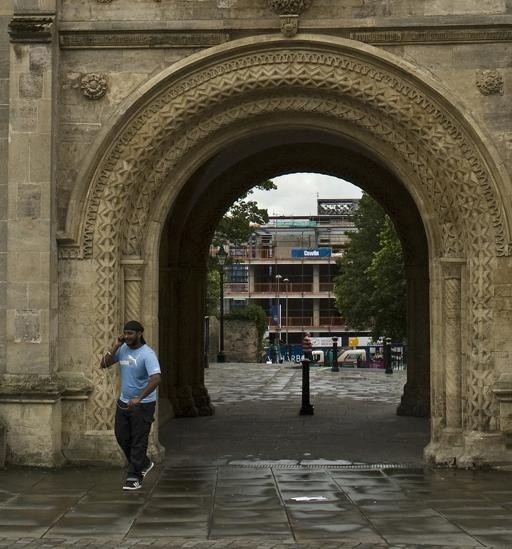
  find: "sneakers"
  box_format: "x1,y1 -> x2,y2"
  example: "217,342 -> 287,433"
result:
141,460 -> 154,477
122,479 -> 142,490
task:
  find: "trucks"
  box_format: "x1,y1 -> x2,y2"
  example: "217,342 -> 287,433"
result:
298,349 -> 395,369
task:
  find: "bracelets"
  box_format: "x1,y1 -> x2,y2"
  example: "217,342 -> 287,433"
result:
107,352 -> 113,358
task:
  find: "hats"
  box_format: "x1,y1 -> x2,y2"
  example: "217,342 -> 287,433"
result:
305,332 -> 310,335
124,321 -> 146,344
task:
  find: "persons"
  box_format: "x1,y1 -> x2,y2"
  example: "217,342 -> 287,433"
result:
98,320 -> 162,491
302,331 -> 317,363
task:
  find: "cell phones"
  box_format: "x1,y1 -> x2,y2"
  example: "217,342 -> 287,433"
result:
120,336 -> 125,342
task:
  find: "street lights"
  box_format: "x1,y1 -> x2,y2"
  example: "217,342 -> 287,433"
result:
215,243 -> 229,362
274,273 -> 282,361
282,278 -> 291,363
